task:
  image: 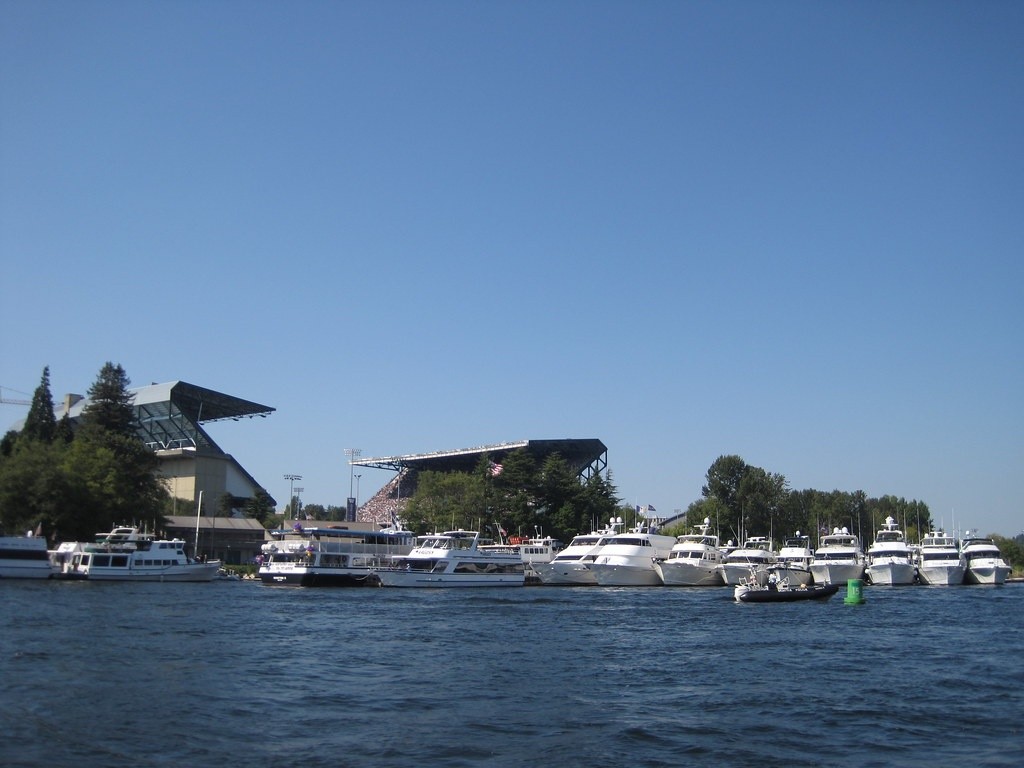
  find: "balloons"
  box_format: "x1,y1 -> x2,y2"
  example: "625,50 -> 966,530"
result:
292,522 -> 301,532
256,554 -> 264,564
305,546 -> 313,558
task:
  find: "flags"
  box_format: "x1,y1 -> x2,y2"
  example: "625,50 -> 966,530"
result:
499,527 -> 507,537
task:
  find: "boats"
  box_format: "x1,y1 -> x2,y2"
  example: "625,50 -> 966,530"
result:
0,507 -> 1009,604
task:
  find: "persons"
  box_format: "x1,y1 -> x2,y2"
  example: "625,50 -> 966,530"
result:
388,562 -> 412,571
